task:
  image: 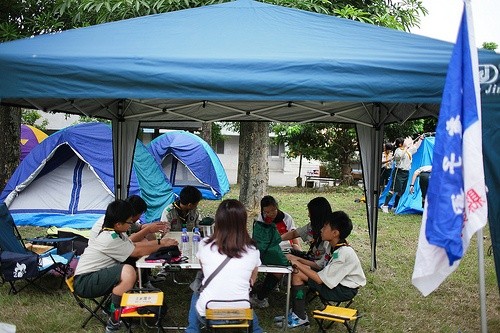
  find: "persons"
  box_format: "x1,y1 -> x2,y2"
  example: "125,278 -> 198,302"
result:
410,166 -> 432,195
73,199 -> 178,333
281,197 -> 332,304
275,211 -> 366,330
381,136 -> 424,208
161,186 -> 202,232
185,199 -> 264,333
249,195 -> 302,308
88,195 -> 169,290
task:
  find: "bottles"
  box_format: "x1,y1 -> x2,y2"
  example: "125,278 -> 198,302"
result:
192,228 -> 200,260
181,228 -> 189,256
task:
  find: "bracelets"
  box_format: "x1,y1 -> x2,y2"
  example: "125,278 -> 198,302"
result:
410,185 -> 414,187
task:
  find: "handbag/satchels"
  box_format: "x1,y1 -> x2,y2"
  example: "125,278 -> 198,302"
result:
145,244 -> 189,263
190,270 -> 206,295
251,219 -> 290,265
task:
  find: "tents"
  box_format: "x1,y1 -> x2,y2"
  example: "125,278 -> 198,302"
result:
21,124 -> 49,161
0,122 -> 176,229
147,129 -> 230,200
0,0 -> 500,292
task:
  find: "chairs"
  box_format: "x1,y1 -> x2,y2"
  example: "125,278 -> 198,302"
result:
199,299 -> 254,333
65,271 -> 114,328
120,288 -> 164,333
0,202 -> 77,295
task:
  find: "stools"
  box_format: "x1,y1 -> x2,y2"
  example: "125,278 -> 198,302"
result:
305,282 -> 362,333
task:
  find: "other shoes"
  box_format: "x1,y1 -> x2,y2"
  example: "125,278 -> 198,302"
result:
259,298 -> 269,308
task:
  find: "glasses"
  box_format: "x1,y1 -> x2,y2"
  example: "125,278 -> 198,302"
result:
126,220 -> 135,227
264,208 -> 277,216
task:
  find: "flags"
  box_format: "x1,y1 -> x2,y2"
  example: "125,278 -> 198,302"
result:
412,0 -> 488,297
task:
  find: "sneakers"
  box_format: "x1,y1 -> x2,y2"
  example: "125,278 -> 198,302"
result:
274,308 -> 310,330
101,309 -> 108,316
106,317 -> 137,333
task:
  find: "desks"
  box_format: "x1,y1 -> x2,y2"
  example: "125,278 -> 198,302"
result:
135,232 -> 293,333
